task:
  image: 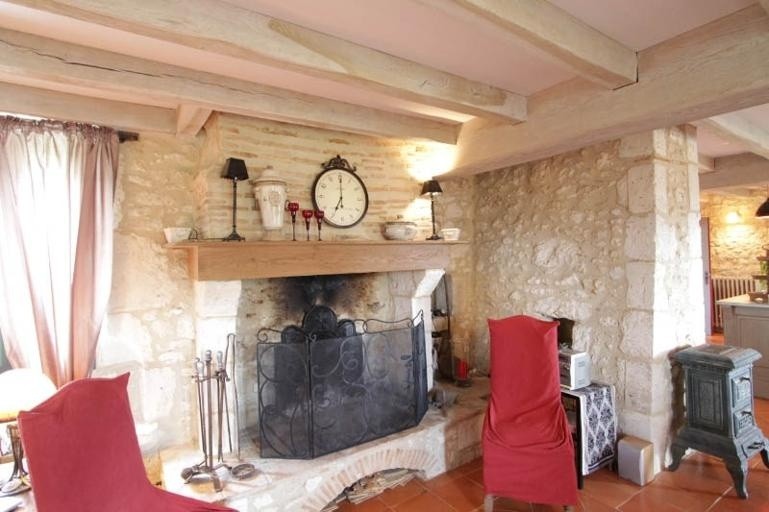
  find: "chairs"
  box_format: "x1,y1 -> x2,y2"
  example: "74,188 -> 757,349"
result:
478,312 -> 579,511
13,366 -> 248,512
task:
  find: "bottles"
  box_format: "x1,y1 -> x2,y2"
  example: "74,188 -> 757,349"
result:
451,335 -> 473,383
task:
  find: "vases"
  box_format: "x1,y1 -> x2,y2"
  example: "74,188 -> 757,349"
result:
440,227 -> 461,242
162,226 -> 193,244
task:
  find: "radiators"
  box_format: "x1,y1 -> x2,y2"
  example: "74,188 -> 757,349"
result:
711,276 -> 760,329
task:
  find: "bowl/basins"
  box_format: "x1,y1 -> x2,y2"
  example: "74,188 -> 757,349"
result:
164,226 -> 193,242
381,214 -> 419,241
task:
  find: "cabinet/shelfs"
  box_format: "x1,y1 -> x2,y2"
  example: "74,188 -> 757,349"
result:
560,381 -> 617,490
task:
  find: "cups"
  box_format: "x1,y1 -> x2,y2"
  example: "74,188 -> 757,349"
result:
437,228 -> 460,240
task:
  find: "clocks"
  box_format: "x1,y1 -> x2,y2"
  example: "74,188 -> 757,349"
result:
311,153 -> 370,230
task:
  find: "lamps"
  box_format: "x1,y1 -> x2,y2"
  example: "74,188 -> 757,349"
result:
753,196 -> 769,219
219,157 -> 249,241
1,363 -> 61,498
421,180 -> 446,242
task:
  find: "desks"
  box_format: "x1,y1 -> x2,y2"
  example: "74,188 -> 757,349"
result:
714,289 -> 768,401
